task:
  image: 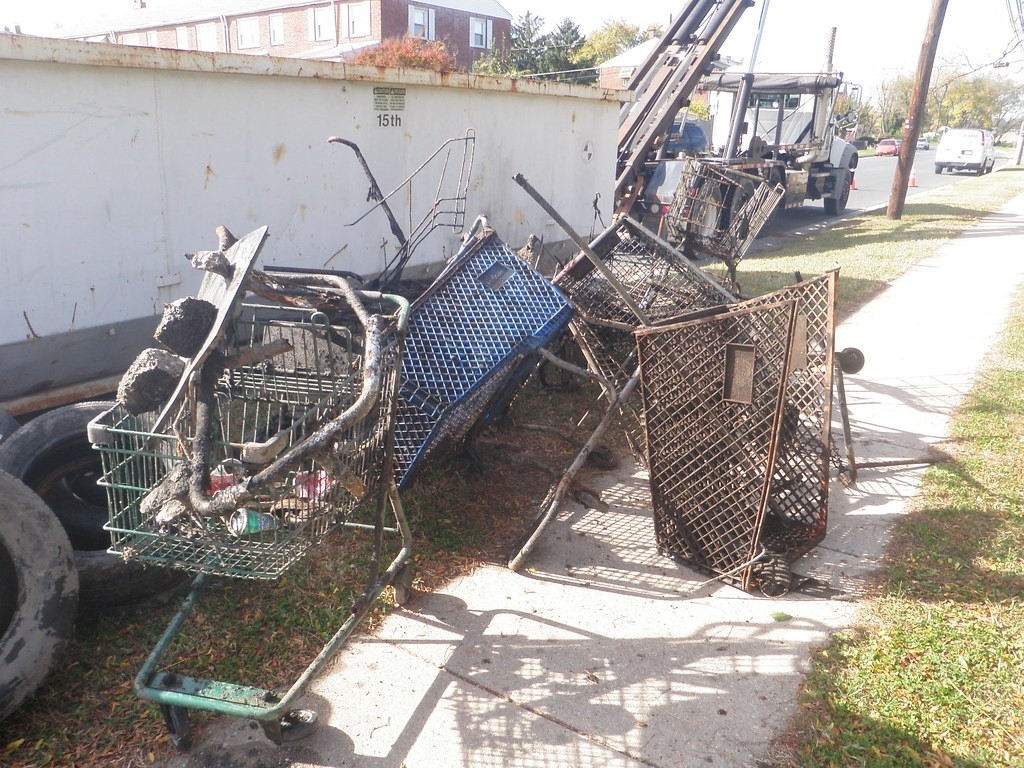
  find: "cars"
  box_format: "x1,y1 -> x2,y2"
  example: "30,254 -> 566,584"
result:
916,138 -> 930,146
874,138 -> 902,156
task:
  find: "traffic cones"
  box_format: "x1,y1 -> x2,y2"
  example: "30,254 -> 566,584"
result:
907,166 -> 918,187
850,177 -> 859,193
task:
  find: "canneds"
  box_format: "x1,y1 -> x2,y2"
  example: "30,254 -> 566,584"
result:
200,458 -> 343,536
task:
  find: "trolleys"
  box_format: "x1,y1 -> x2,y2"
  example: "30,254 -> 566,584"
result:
67,134 -> 870,740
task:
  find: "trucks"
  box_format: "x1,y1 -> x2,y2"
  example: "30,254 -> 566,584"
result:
638,70 -> 864,224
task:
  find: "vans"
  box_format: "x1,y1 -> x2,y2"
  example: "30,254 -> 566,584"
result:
934,128 -> 997,175
